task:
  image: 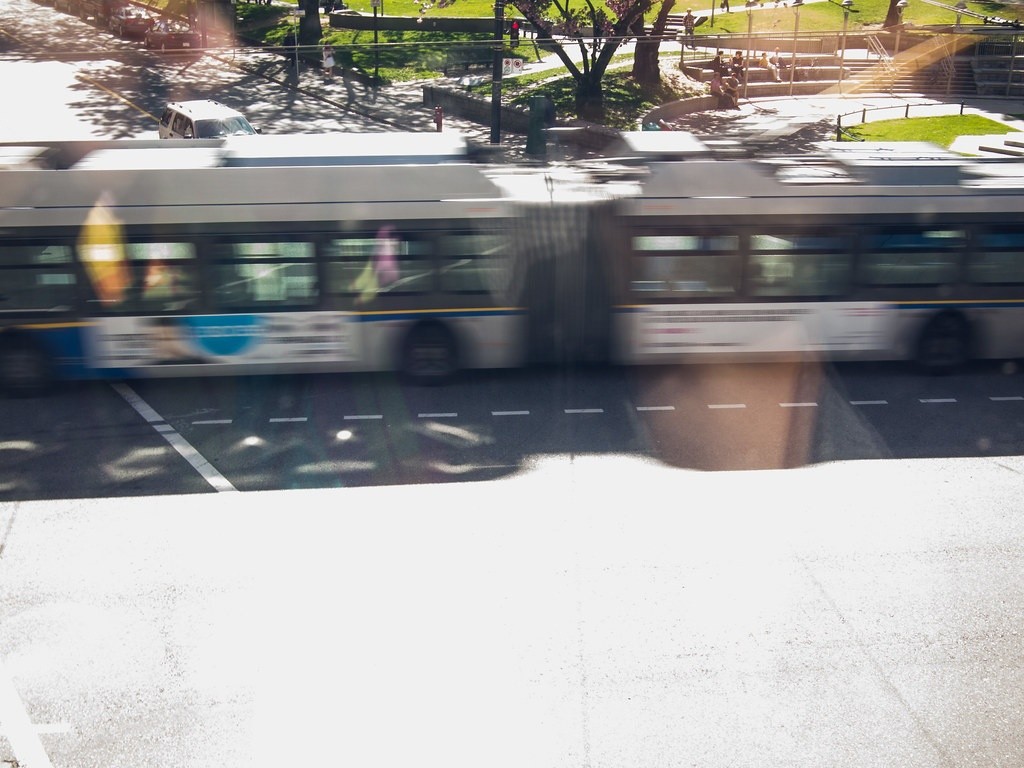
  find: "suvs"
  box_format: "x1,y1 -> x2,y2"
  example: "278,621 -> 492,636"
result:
159,98 -> 262,141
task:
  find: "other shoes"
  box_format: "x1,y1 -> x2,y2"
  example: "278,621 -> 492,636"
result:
776,78 -> 782,82
735,106 -> 740,110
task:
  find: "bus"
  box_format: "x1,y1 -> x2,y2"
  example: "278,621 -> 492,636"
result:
0,133 -> 1024,385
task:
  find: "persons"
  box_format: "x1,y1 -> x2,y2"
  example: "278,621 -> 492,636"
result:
283,28 -> 299,68
322,41 -> 336,81
681,0 -> 791,110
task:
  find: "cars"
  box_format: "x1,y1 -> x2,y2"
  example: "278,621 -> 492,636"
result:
54,0 -> 201,54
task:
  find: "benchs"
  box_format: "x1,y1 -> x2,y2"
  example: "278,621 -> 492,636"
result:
683,55 -> 860,98
443,47 -> 494,75
971,55 -> 1024,95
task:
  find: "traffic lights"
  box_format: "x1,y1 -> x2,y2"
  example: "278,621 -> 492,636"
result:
510,20 -> 521,48
